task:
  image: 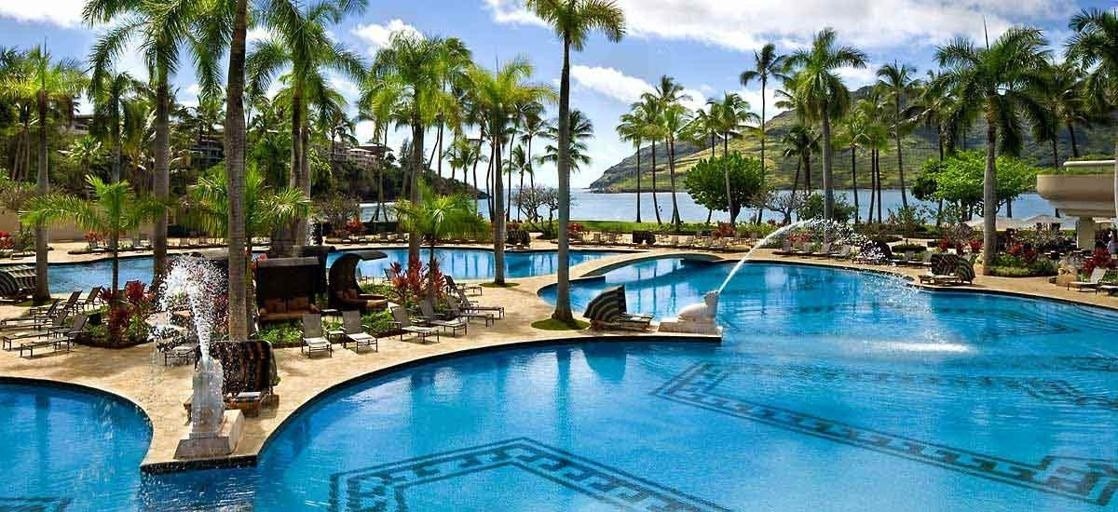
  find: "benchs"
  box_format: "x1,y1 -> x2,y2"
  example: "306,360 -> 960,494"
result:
181,341 -> 280,424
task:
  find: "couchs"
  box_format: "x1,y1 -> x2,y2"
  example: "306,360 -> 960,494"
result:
255,256 -> 321,329
328,250 -> 388,315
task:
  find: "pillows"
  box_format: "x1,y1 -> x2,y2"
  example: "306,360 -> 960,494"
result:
263,295 -> 310,312
336,289 -> 358,300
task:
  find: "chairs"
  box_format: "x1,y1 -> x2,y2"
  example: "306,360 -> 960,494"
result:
87,228 -> 209,255
342,225 -> 407,244
1067,266 -> 1107,292
164,341 -> 201,371
1096,285 -> 1118,295
569,229 -> 758,249
889,254 -> 975,286
0,283 -> 102,358
584,285 -> 655,332
340,310 -> 377,353
299,313 -> 332,358
382,266 -> 505,344
775,240 -> 849,261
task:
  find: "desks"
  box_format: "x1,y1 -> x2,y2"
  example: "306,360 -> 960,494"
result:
326,330 -> 345,349
